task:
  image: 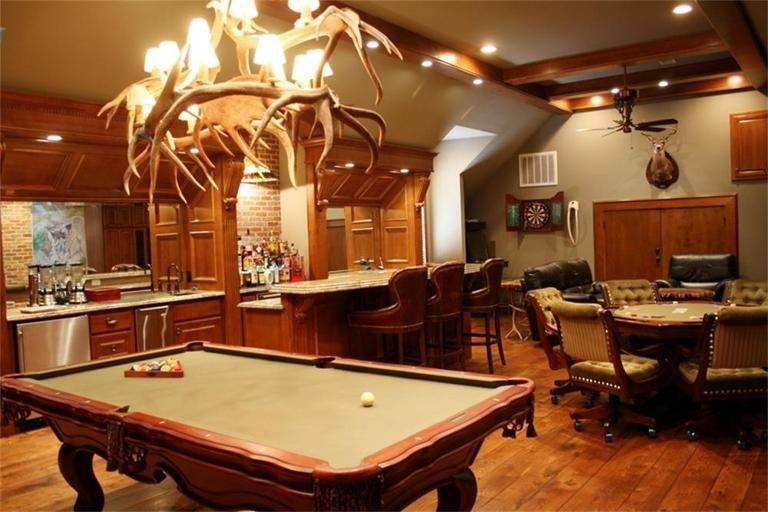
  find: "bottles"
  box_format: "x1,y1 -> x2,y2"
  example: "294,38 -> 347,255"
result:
236,226 -> 304,285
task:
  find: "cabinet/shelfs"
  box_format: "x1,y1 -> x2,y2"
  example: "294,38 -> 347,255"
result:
730,109 -> 767,182
89,307 -> 137,360
172,296 -> 226,345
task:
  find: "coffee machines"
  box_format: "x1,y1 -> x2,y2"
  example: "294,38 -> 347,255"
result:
26,259 -> 88,308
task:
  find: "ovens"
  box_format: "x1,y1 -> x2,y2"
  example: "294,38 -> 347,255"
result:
16,315 -> 91,374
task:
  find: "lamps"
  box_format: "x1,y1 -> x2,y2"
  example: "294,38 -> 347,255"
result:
96,0 -> 404,204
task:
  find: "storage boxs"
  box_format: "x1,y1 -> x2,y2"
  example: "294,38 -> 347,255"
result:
86,288 -> 121,302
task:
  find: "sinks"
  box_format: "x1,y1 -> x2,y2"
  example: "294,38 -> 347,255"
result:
173,292 -> 196,296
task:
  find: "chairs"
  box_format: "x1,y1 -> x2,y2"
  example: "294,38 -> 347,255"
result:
348,258 -> 506,375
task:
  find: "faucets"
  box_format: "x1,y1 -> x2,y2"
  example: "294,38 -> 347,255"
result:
145,264 -> 154,291
167,263 -> 178,291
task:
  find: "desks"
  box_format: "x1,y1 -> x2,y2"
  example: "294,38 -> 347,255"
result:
0,341 -> 536,512
501,278 -> 531,343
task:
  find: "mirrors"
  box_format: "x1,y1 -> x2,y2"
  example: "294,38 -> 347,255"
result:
32,201 -> 152,289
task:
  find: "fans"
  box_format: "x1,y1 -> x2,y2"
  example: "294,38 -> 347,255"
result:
576,64 -> 678,137
